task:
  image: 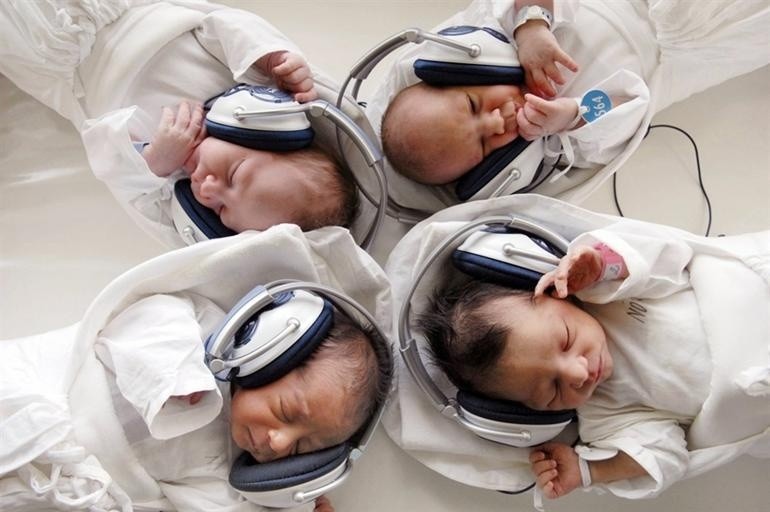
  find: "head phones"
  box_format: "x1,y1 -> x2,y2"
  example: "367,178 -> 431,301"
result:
203,278 -> 394,508
397,213 -> 576,449
170,84 -> 388,254
335,25 -> 545,227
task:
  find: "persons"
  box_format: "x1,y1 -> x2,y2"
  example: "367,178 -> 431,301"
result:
93,279 -> 393,512
74,0 -> 388,251
397,215 -> 712,501
334,0 -> 659,225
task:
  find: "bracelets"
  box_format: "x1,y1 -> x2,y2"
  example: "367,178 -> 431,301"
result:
577,457 -> 594,488
595,243 -> 625,282
512,5 -> 553,39
566,96 -> 586,132
